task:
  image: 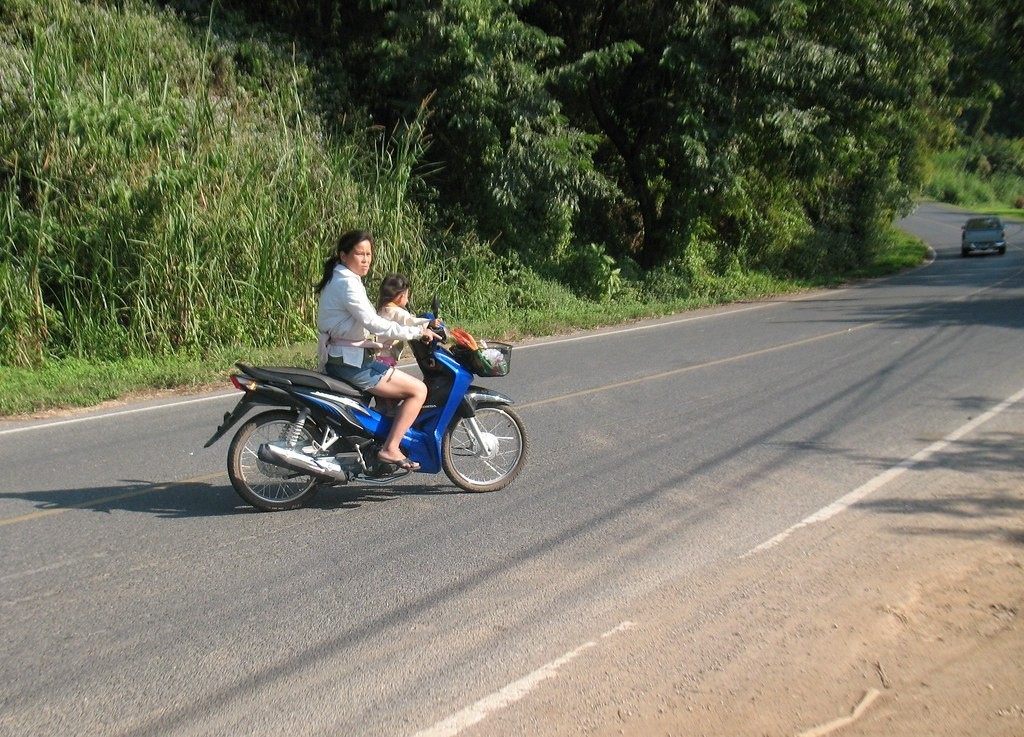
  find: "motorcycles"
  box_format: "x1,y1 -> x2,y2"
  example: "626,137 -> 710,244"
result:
205,311 -> 531,514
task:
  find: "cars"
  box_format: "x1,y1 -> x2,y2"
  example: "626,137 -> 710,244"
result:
958,214 -> 1006,255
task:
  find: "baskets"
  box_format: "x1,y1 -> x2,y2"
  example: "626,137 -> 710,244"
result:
451,342 -> 513,378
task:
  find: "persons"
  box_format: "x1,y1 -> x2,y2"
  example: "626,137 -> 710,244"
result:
316,230 -> 442,471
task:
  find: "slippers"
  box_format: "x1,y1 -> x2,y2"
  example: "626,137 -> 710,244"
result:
375,455 -> 421,471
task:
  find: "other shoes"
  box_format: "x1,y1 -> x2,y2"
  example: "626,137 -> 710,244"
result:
371,406 -> 396,417
